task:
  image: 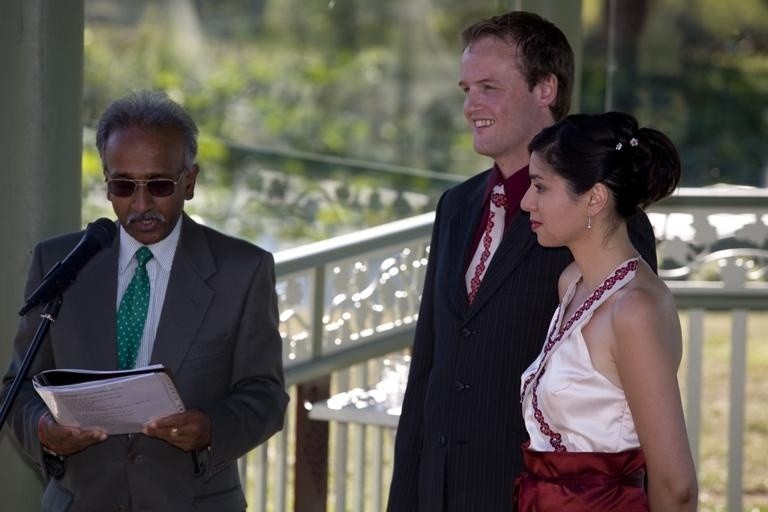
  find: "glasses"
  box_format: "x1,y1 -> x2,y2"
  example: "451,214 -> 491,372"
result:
103,170 -> 184,198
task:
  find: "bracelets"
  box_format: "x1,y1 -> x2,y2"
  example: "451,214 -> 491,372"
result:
42,445 -> 65,463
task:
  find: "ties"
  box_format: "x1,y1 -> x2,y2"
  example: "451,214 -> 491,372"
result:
464,184 -> 507,307
115,247 -> 153,371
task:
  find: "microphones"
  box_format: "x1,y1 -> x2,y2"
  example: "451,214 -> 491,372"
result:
18,217 -> 118,316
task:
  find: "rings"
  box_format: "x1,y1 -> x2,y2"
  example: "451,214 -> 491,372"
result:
170,426 -> 178,444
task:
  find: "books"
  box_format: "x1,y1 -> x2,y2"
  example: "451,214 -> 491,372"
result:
31,362 -> 186,437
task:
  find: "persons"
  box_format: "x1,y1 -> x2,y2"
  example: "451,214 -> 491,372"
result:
518,110 -> 697,512
1,91 -> 291,512
385,10 -> 657,512
